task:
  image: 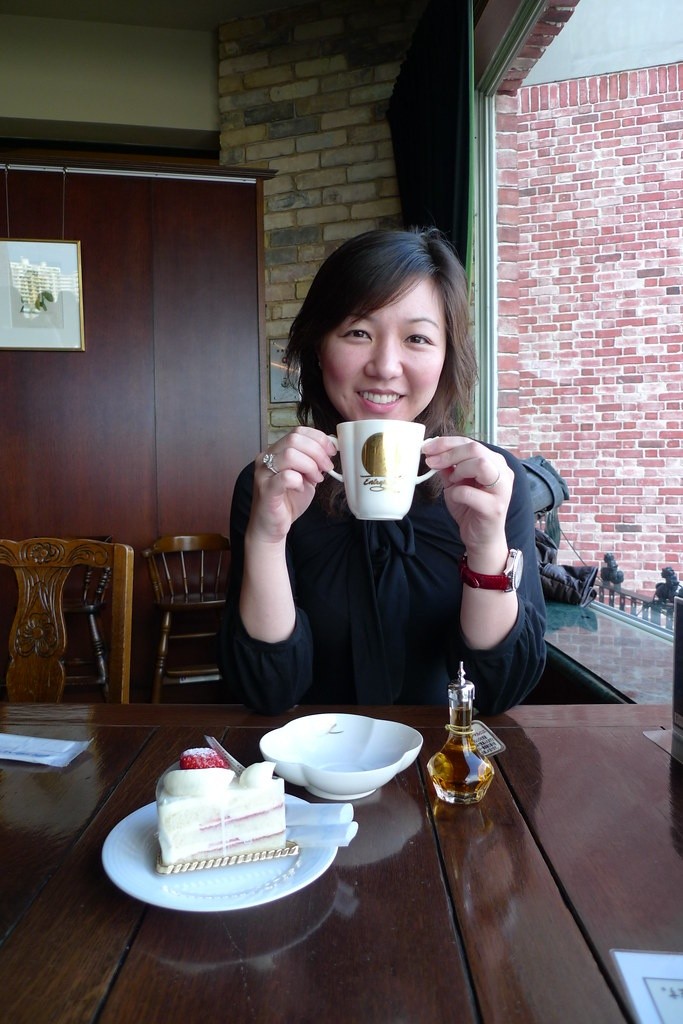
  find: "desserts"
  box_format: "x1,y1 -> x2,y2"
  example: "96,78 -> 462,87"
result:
154,734 -> 300,875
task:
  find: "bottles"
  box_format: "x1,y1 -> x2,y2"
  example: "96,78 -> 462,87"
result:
427,659 -> 495,806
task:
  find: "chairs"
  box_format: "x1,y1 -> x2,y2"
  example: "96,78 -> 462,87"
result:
141,526 -> 235,699
0,539 -> 139,703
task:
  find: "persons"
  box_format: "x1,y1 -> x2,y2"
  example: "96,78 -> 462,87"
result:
216,230 -> 547,717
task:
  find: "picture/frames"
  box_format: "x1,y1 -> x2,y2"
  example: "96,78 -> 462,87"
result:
2,238 -> 84,352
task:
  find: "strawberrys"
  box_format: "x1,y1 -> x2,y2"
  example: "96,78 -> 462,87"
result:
180,748 -> 230,769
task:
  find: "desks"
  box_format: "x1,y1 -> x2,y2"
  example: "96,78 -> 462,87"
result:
1,701 -> 683,1024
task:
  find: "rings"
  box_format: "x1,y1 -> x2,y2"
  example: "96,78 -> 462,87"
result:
261,452 -> 278,474
482,468 -> 502,488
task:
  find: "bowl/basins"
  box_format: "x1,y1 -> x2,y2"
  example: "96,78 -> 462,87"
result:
258,712 -> 424,800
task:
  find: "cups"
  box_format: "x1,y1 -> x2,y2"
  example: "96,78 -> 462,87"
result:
322,419 -> 442,522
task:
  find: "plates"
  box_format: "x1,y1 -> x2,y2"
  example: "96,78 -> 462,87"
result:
100,795 -> 339,912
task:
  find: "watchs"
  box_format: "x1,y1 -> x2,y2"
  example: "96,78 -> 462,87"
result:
460,548 -> 523,593
